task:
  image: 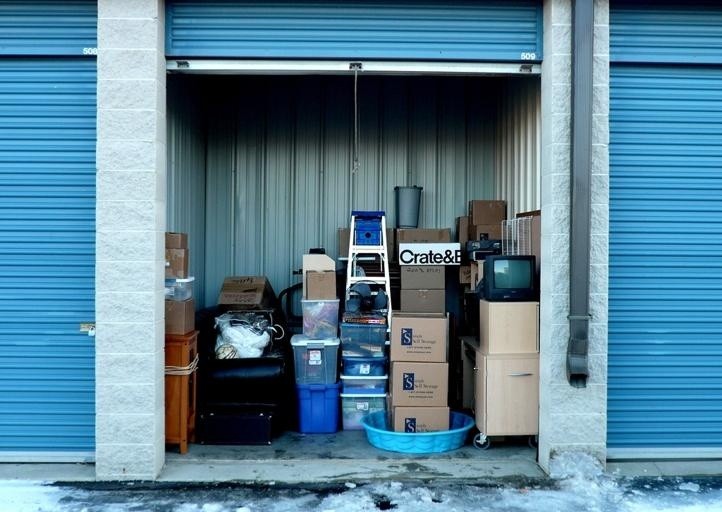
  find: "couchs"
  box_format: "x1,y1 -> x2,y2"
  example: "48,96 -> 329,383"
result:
197,306 -> 294,447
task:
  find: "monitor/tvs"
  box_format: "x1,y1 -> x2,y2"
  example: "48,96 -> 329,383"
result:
484,255 -> 536,302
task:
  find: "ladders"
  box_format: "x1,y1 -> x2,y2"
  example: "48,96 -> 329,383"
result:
345,210 -> 392,347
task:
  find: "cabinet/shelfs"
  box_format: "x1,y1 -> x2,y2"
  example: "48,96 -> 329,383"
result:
460,300 -> 539,450
165,330 -> 199,454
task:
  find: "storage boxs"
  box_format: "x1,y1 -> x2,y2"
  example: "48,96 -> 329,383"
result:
400,289 -> 446,313
457,215 -> 468,248
340,358 -> 388,376
296,382 -> 341,434
396,229 -> 451,243
339,374 -> 389,395
390,316 -> 447,362
165,248 -> 187,278
217,275 -> 278,309
393,407 -> 450,433
401,266 -> 445,289
470,225 -> 503,241
338,227 -> 395,259
290,333 -> 341,384
166,232 -> 187,248
339,322 -> 387,358
392,362 -> 449,407
300,296 -> 341,339
469,200 -> 506,225
307,271 -> 337,300
165,277 -> 195,302
338,394 -> 386,431
165,301 -> 195,335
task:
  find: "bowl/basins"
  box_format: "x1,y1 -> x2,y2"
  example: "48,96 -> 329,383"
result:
357,410 -> 475,454
